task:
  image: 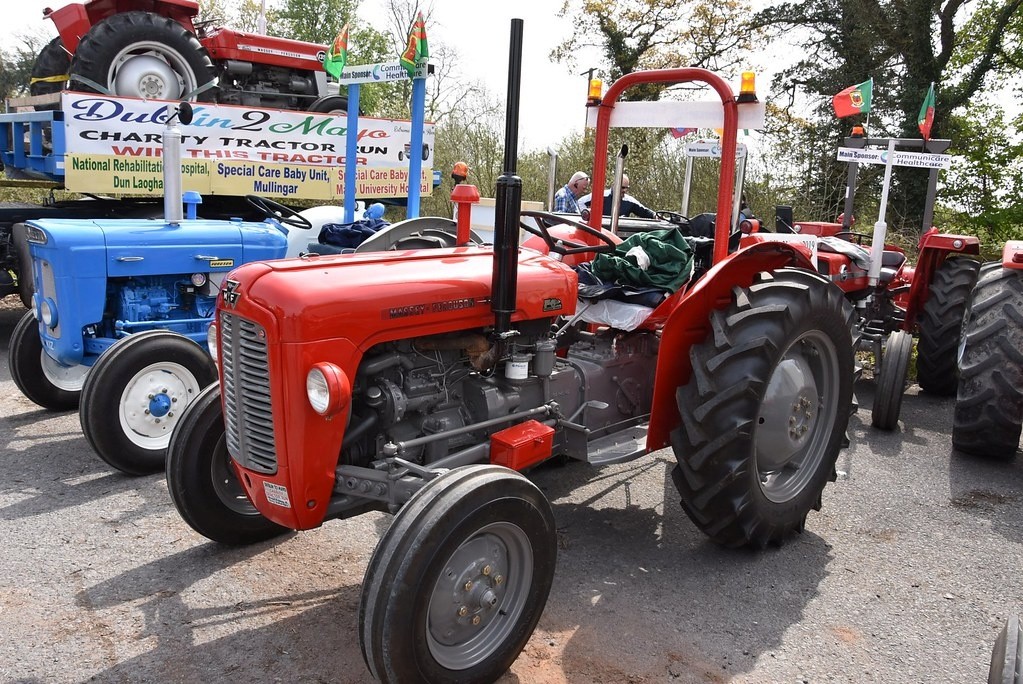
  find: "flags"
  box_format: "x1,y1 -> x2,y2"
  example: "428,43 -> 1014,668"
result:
322,22 -> 349,81
833,80 -> 871,117
669,128 -> 695,139
400,11 -> 429,79
918,85 -> 936,140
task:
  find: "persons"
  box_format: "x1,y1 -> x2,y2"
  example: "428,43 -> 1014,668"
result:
555,171 -> 590,214
602,174 -> 660,219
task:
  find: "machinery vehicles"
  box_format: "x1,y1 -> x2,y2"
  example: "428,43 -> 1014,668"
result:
162,16 -> 864,683
950,238 -> 1023,459
732,79 -> 986,430
8,55 -> 484,477
30,0 -> 366,127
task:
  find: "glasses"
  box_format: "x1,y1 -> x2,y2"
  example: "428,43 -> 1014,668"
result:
575,175 -> 592,183
622,186 -> 629,189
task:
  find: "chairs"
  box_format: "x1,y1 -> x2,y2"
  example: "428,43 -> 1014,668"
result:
878,251 -> 905,282
680,213 -> 740,283
